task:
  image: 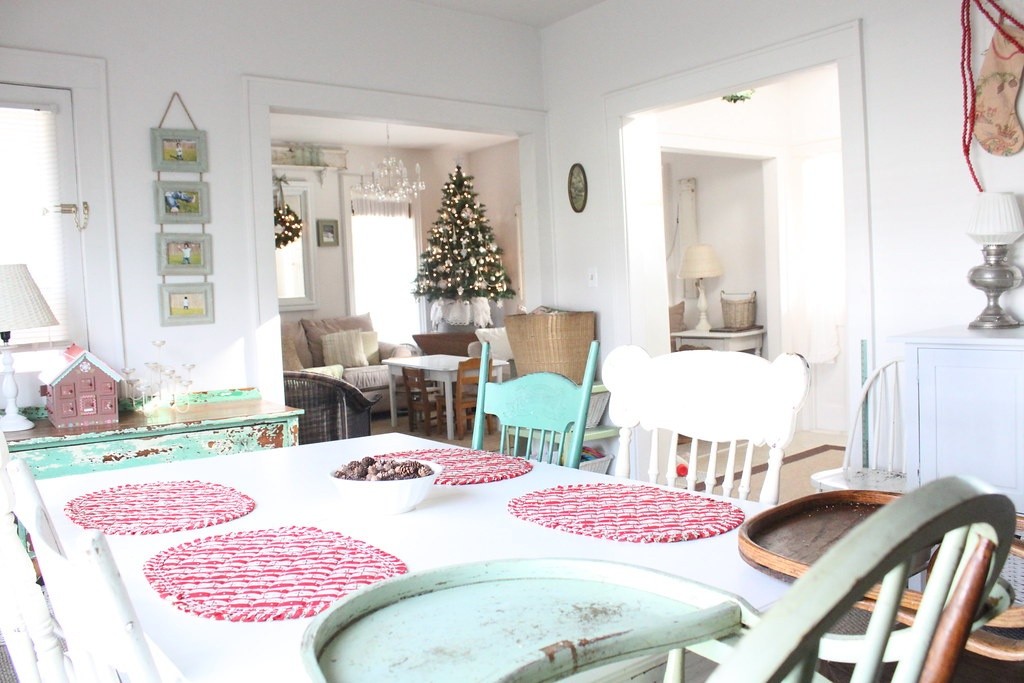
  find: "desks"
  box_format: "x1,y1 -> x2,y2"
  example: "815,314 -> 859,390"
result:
34,433 -> 791,683
382,353 -> 515,441
0,388 -> 304,558
670,329 -> 767,353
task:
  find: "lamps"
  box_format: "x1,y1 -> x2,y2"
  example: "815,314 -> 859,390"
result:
967,191 -> 1024,328
676,244 -> 722,334
0,264 -> 60,432
351,124 -> 426,203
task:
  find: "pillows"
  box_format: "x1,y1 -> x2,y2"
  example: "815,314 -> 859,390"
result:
360,331 -> 380,364
281,336 -> 304,371
475,326 -> 514,360
320,328 -> 369,368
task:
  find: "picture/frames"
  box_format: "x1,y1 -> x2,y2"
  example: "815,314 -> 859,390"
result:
317,219 -> 339,247
150,127 -> 215,326
568,163 -> 588,213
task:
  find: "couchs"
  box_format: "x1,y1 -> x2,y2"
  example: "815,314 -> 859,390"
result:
281,312 -> 413,415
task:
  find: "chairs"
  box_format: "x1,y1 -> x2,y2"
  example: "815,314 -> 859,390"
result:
662,473 -> 1024,683
809,357 -> 903,492
0,432 -> 160,683
668,301 -> 686,352
283,372 -> 383,445
404,340 -> 810,507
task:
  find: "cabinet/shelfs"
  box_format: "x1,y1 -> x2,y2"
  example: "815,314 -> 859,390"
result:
496,380 -> 620,463
890,323 -> 1024,514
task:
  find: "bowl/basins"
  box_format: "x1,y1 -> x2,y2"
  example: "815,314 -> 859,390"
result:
328,459 -> 445,516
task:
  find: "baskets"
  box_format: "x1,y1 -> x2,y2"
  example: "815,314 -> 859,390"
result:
720,291 -> 756,329
504,311 -> 596,383
